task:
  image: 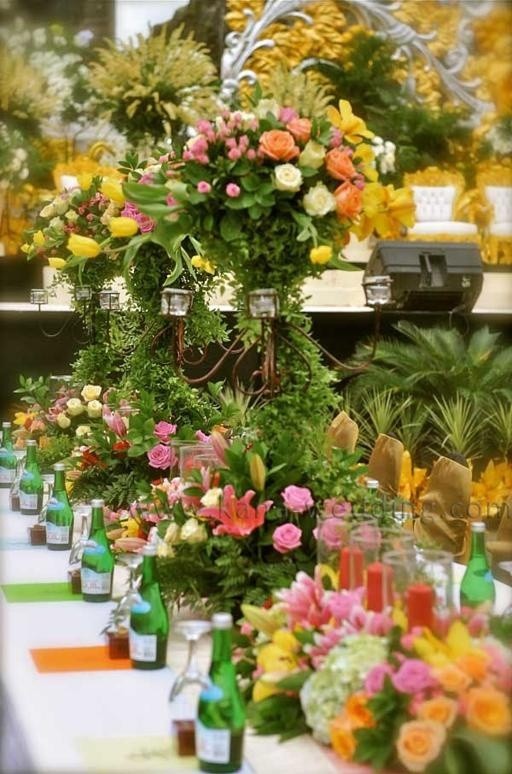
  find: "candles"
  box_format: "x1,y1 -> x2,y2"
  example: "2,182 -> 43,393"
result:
338,548 -> 361,590
188,456 -> 199,473
407,586 -> 435,634
211,474 -> 222,489
367,563 -> 393,610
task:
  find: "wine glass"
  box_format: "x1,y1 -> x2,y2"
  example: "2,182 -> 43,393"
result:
69,502 -> 92,566
117,555 -> 144,617
38,479 -> 54,523
10,453 -> 25,507
168,617 -> 208,707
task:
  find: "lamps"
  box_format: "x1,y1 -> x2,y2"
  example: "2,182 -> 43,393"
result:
27,284 -> 92,341
150,275 -> 392,406
93,292 -> 163,347
359,240 -> 484,313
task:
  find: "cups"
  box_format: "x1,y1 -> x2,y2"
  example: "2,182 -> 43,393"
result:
117,409 -> 140,432
101,403 -> 122,428
381,547 -> 452,630
313,510 -> 379,592
350,523 -> 411,603
192,454 -> 220,482
178,442 -> 212,479
168,437 -> 196,477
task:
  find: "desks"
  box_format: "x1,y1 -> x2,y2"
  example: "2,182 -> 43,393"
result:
0,439 -> 511,774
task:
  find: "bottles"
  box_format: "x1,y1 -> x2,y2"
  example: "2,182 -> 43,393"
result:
44,462 -> 71,551
82,499 -> 114,602
19,437 -> 41,514
193,612 -> 248,773
365,480 -> 380,503
127,543 -> 167,669
460,519 -> 497,617
1,421 -> 17,486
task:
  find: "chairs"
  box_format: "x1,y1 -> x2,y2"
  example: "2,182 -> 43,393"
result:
485,494 -> 509,595
476,162 -> 512,265
404,166 -> 489,267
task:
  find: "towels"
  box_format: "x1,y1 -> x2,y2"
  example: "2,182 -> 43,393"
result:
409,455 -> 468,561
327,413 -> 358,458
370,432 -> 402,497
